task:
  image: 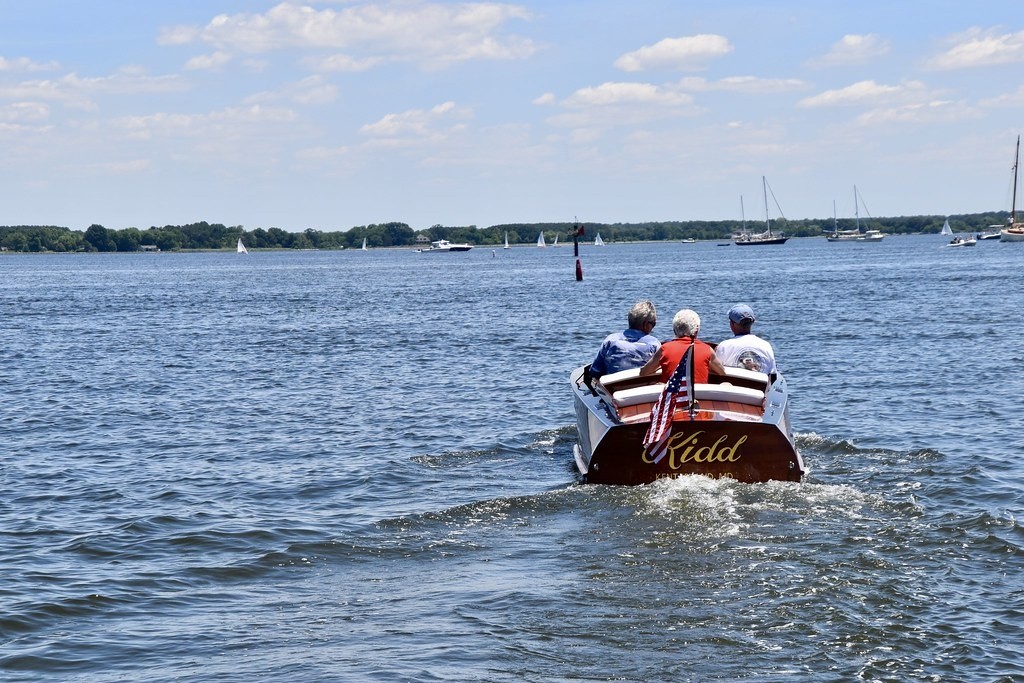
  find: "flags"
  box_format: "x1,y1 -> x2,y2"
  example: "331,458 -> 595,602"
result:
642,343 -> 694,447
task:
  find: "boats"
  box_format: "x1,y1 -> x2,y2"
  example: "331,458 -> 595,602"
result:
412,239 -> 474,252
945,239 -> 977,247
976,224 -> 1006,241
681,238 -> 696,243
568,340 -> 805,487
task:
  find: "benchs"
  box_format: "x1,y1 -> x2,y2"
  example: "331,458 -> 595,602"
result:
599,364 -> 769,417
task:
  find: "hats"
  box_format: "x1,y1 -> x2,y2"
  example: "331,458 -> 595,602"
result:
727,304 -> 756,324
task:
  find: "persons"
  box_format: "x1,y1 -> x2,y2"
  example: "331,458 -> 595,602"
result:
715,303 -> 777,377
640,308 -> 727,386
588,300 -> 663,380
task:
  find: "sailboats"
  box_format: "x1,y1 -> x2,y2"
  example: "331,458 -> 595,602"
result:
552,233 -> 561,248
594,232 -> 606,246
503,231 -> 512,249
940,218 -> 954,236
537,230 -> 546,248
822,184 -> 886,242
999,134 -> 1024,243
731,175 -> 794,246
362,237 -> 368,252
236,237 -> 248,255
